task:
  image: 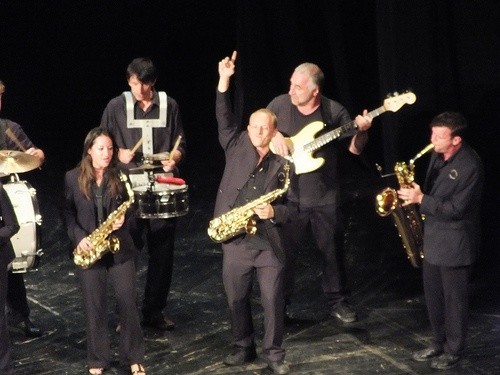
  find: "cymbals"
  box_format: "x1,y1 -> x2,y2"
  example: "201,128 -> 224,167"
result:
129,163 -> 163,170
0,149 -> 41,173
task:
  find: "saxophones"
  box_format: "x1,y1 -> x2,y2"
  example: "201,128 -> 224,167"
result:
72,169 -> 134,270
208,161 -> 290,244
375,143 -> 435,268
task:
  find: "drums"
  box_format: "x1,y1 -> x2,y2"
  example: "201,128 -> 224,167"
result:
133,182 -> 190,219
2,180 -> 37,272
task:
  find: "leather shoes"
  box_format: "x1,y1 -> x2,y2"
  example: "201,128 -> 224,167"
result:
15,318 -> 42,336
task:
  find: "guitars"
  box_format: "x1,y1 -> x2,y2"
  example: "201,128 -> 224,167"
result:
269,90 -> 416,175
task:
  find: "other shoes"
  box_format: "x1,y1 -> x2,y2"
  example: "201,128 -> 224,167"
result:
88,367 -> 104,375
267,360 -> 290,375
331,304 -> 357,323
411,344 -> 470,369
143,310 -> 176,329
129,363 -> 146,375
116,311 -> 143,334
223,350 -> 255,363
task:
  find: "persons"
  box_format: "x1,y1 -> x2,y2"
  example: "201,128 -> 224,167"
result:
61,127 -> 148,375
99,56 -> 186,333
397,111 -> 482,370
0,81 -> 44,375
213,51 -> 300,375
261,63 -> 372,323
